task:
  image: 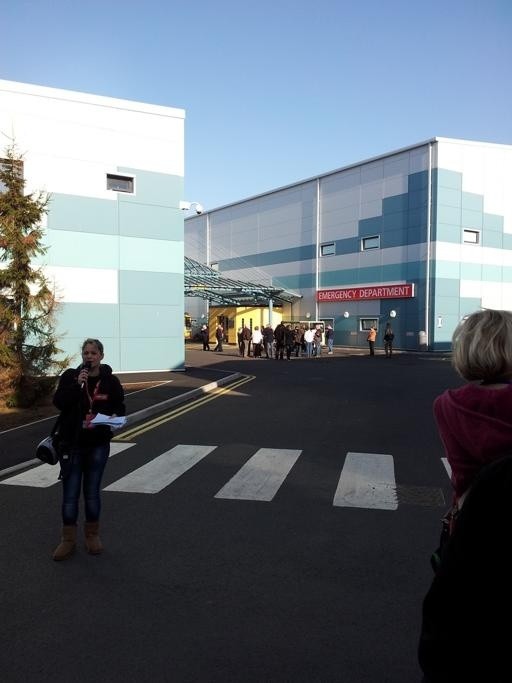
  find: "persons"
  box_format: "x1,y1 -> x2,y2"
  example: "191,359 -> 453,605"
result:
433,308 -> 512,500
35,338 -> 127,561
383,323 -> 394,357
367,326 -> 376,358
201,320 -> 335,362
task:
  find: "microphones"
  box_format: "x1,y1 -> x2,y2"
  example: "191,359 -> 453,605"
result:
81,361 -> 91,390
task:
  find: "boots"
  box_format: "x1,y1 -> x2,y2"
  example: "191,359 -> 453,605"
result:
51,525 -> 76,560
84,521 -> 103,554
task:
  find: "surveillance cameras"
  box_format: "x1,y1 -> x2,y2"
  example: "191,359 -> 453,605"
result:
196,205 -> 203,215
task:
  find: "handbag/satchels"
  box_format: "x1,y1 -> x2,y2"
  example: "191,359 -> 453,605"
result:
35,433 -> 59,465
